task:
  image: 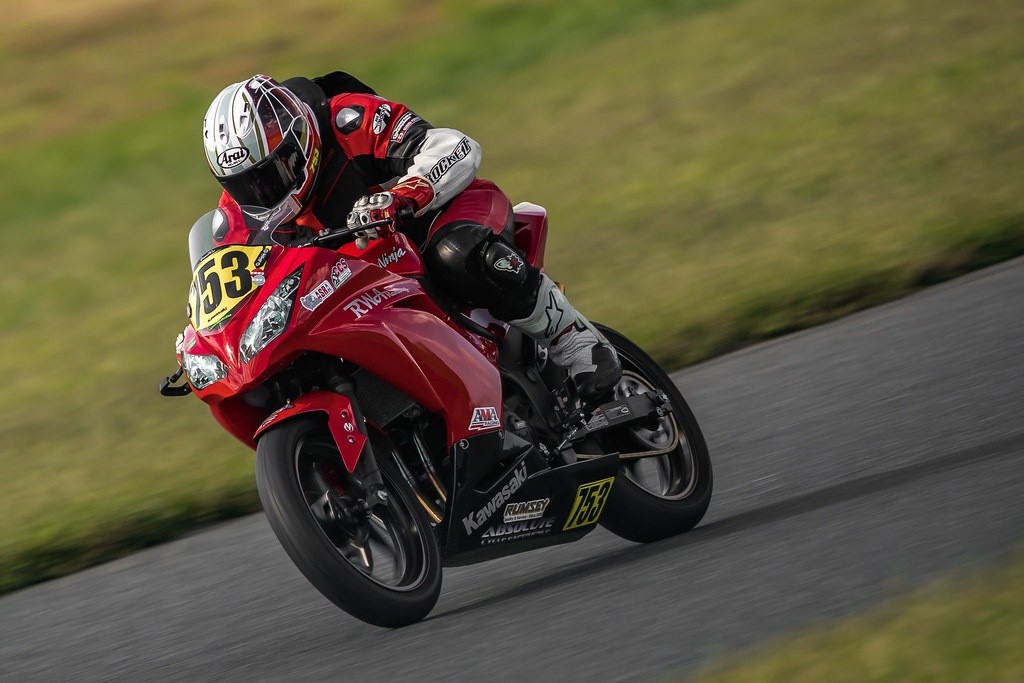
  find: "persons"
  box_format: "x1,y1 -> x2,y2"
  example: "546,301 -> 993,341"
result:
176,71 -> 622,402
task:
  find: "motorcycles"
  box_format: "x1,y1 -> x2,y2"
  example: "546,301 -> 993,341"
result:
158,200 -> 714,628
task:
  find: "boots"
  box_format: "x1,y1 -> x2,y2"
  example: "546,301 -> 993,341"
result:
508,275 -> 621,401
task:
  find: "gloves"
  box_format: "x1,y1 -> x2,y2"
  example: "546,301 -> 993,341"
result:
345,192 -> 413,249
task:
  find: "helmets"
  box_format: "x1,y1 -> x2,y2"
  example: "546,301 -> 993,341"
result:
202,74 -> 322,225
